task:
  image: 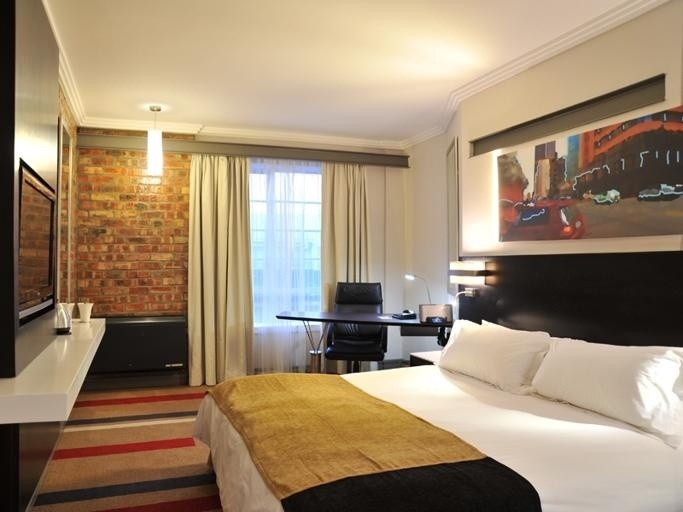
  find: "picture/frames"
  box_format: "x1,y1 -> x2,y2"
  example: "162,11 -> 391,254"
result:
445,136 -> 457,298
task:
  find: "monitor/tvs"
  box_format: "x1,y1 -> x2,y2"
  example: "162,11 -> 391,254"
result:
17,164 -> 61,320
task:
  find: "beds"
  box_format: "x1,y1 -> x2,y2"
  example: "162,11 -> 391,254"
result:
193,249 -> 682,512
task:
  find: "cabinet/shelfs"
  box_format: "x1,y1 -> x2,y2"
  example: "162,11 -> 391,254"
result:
87,314 -> 187,379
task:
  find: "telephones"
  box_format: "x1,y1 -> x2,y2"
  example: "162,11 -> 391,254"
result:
392,310 -> 416,319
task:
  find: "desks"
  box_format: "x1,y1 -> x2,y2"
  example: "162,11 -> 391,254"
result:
275,310 -> 453,374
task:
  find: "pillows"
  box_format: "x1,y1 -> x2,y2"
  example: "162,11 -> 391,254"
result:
531,336 -> 682,448
480,317 -> 551,386
440,320 -> 550,393
639,377 -> 682,452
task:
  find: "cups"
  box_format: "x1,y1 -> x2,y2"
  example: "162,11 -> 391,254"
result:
77,303 -> 94,323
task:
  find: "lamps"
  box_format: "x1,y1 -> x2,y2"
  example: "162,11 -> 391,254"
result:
146,103 -> 166,179
404,272 -> 432,305
449,260 -> 485,285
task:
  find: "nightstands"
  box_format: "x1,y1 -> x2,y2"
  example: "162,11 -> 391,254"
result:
409,350 -> 441,368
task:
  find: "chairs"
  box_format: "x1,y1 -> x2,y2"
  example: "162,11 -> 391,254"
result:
324,278 -> 387,372
